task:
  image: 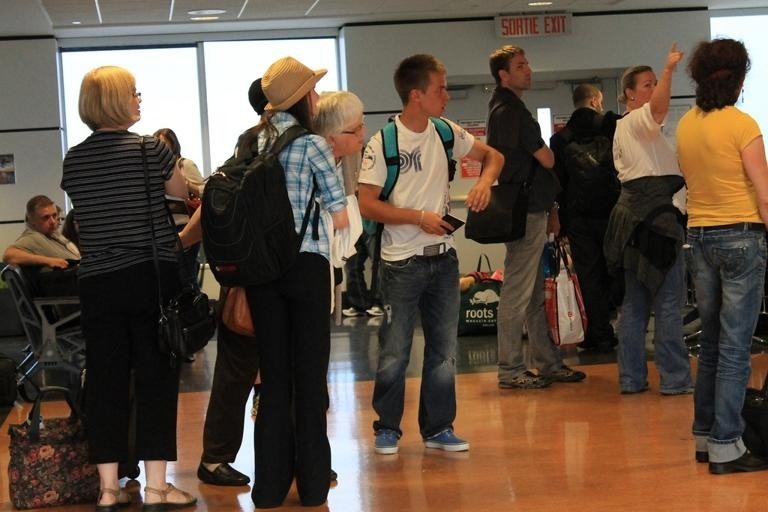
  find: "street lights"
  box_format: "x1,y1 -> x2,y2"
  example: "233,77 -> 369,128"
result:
457,255 -> 503,336
7,387 -> 101,509
465,181 -> 530,244
545,240 -> 587,345
159,284 -> 218,367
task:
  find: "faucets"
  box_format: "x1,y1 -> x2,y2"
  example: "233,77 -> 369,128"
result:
98,486 -> 133,512
142,484 -> 198,512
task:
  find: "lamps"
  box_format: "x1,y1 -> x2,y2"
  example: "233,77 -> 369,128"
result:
0,263 -> 86,389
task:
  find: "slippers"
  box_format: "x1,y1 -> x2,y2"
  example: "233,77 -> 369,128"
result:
202,125 -> 321,287
558,115 -> 615,201
354,115 -> 457,260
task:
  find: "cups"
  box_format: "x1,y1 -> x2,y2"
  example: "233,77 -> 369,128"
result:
131,93 -> 140,102
340,116 -> 366,134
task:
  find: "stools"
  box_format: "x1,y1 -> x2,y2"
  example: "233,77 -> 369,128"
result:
693,223 -> 768,233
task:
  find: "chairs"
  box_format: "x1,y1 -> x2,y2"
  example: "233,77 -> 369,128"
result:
620,382 -> 648,394
425,429 -> 469,452
342,306 -> 361,316
366,306 -> 390,315
660,382 -> 695,395
374,430 -> 399,456
537,364 -> 586,382
498,370 -> 551,389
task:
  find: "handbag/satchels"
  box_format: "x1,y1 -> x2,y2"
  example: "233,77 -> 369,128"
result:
418,209 -> 425,229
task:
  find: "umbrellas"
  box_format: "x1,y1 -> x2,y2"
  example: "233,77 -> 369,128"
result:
696,451 -> 709,462
710,450 -> 768,474
197,462 -> 250,486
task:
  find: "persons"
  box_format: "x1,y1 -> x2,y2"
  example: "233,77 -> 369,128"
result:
485,44 -> 587,391
232,55 -> 350,507
52,205 -> 81,257
153,127 -> 206,282
248,76 -> 269,125
177,89 -> 368,486
341,234 -> 385,317
58,65 -> 199,510
604,40 -> 696,397
250,370 -> 262,417
675,36 -> 767,479
549,80 -> 631,353
3,194 -> 83,333
356,53 -> 506,457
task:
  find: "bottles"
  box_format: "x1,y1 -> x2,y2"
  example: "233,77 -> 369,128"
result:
262,58 -> 328,111
